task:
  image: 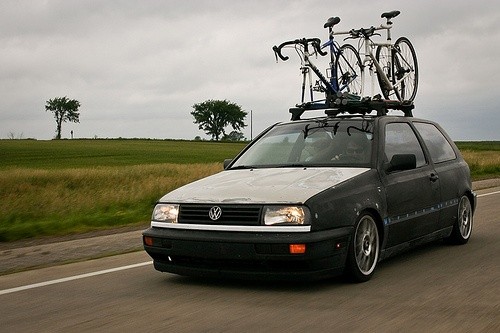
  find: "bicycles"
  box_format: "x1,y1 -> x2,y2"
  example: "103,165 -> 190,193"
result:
342,10 -> 420,107
272,16 -> 365,108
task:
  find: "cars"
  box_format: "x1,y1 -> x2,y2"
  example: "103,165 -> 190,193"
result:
140,96 -> 480,288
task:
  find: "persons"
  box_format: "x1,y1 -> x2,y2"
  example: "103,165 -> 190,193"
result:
330,139 -> 368,162
304,132 -> 334,163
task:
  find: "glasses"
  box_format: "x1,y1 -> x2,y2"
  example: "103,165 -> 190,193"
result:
347,148 -> 363,154
